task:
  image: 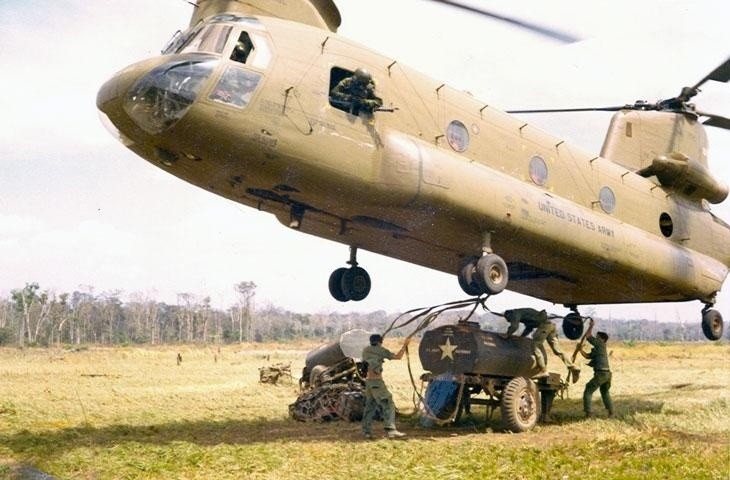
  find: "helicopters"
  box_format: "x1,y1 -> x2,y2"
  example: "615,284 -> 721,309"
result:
95,1 -> 730,347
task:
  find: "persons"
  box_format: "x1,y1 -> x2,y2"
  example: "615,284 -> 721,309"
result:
577,319 -> 614,419
497,307 -> 580,384
332,68 -> 383,116
355,333 -> 407,439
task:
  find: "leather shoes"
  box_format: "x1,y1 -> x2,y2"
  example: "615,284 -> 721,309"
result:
388,430 -> 407,439
364,432 -> 377,439
